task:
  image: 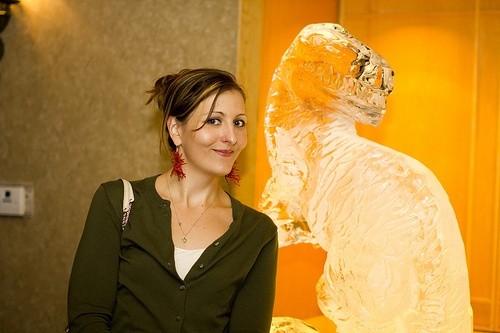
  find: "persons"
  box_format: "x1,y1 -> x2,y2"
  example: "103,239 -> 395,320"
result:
66,68 -> 279,333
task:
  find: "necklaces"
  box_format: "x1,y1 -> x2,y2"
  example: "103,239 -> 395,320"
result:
165,172 -> 221,244
